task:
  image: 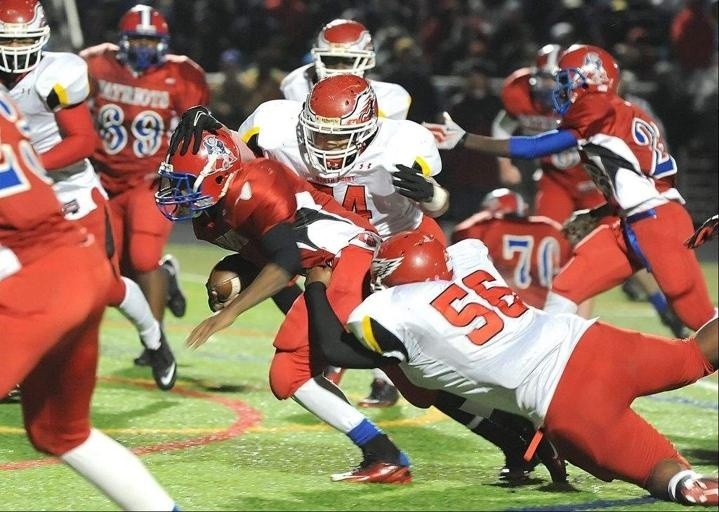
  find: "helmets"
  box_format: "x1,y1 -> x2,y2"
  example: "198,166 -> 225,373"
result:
368,230 -> 454,293
118,2 -> 169,56
481,187 -> 529,220
498,43 -> 622,128
302,15 -> 379,173
0,0 -> 50,67
158,126 -> 242,211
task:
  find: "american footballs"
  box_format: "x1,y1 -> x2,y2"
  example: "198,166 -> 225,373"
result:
209,261 -> 255,304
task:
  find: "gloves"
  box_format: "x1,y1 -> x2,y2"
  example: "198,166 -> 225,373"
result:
422,111 -> 468,153
166,106 -> 223,156
390,160 -> 434,204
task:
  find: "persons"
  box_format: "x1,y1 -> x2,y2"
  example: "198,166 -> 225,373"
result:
0,0 -> 719,485
305,229 -> 719,506
0,85 -> 176,510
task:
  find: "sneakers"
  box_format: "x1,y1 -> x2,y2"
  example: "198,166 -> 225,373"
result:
158,252 -> 186,318
357,377 -> 399,408
499,426 -> 567,484
133,328 -> 177,391
329,450 -> 412,485
666,469 -> 719,505
